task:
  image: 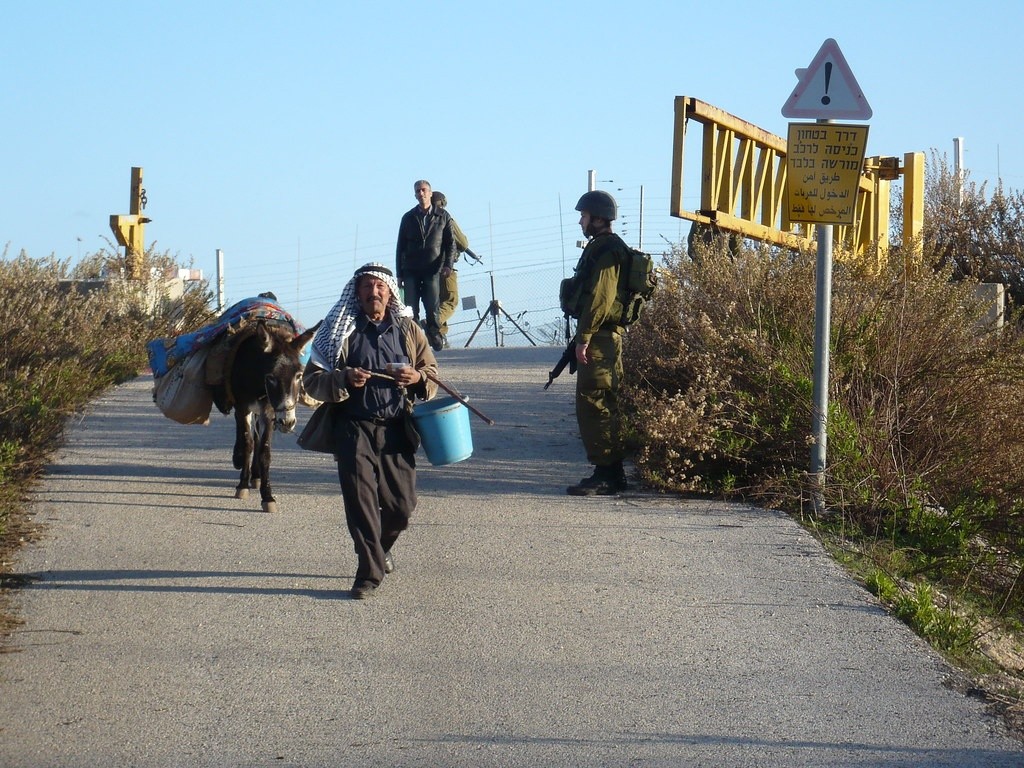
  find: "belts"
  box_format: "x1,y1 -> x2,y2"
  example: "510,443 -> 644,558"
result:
366,414 -> 402,426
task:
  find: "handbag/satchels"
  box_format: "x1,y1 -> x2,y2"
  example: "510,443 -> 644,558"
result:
560,278 -> 584,338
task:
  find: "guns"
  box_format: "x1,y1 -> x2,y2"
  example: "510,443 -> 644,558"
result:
543,333 -> 578,392
464,248 -> 484,265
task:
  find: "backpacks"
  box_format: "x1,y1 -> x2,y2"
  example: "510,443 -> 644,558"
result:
581,232 -> 657,302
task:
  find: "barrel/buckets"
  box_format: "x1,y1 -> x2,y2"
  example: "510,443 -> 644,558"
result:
412,394 -> 473,465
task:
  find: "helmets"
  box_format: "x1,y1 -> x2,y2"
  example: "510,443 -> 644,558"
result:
430,191 -> 447,208
575,191 -> 617,220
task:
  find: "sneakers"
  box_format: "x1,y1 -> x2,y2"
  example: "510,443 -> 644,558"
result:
567,479 -> 617,495
580,470 -> 627,491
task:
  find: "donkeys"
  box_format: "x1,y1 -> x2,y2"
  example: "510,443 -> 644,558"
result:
231,292 -> 324,513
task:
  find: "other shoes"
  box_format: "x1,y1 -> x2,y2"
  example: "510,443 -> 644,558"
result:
351,584 -> 373,599
431,332 -> 444,351
421,318 -> 432,346
442,338 -> 450,349
384,551 -> 395,574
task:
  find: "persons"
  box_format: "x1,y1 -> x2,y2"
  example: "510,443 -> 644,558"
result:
395,180 -> 469,349
304,262 -> 438,599
564,191 -> 630,495
